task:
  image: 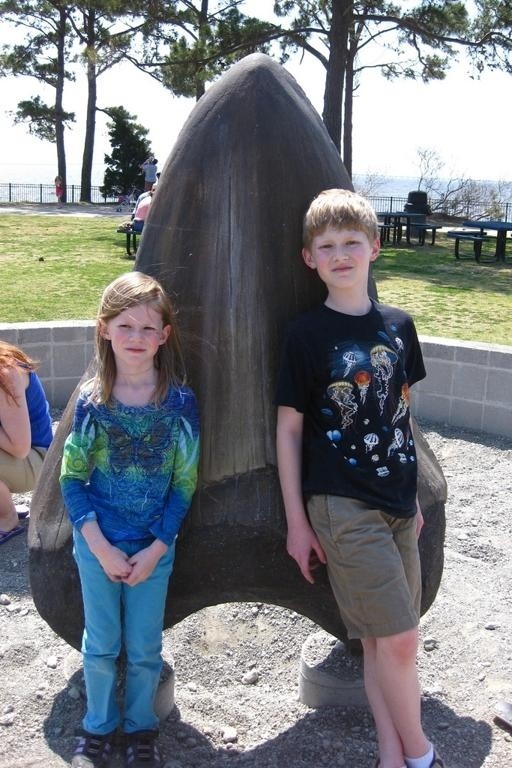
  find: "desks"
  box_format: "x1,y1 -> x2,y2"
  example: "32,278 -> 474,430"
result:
463,220 -> 512,267
375,211 -> 427,246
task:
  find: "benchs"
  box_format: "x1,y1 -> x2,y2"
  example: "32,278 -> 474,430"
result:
117,230 -> 141,258
378,222 -> 489,267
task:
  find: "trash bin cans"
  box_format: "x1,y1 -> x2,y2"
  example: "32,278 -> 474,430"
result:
404,191 -> 431,238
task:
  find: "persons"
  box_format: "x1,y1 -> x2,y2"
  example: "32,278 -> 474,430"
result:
54,176 -> 64,210
1,339 -> 54,544
140,156 -> 158,191
273,189 -> 446,768
130,173 -> 161,233
57,270 -> 202,767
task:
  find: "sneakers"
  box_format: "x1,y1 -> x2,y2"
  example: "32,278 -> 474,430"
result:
125,728 -> 162,768
72,732 -> 113,767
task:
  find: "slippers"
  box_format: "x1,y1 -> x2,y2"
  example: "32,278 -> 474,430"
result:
0,526 -> 25,545
